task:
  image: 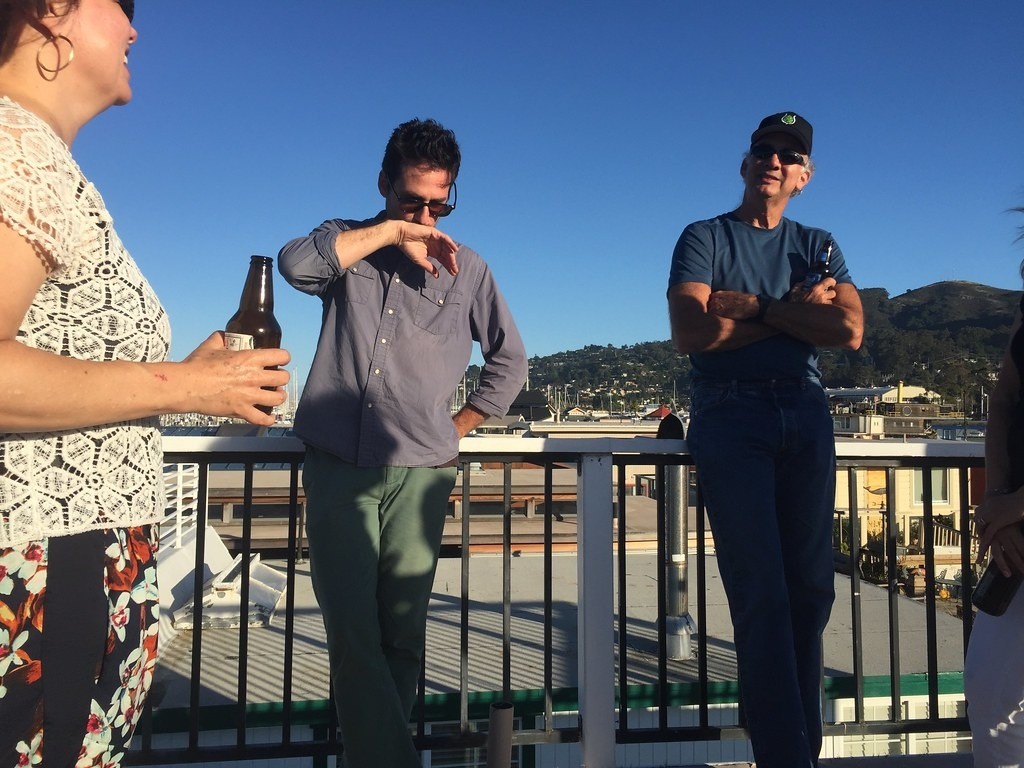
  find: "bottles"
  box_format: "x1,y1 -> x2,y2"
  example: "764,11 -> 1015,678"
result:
223,255 -> 282,416
972,551 -> 1024,616
803,239 -> 834,294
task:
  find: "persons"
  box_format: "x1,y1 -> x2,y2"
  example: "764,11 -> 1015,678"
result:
961,322 -> 1023,768
667,111 -> 865,768
0,0 -> 289,768
277,115 -> 528,767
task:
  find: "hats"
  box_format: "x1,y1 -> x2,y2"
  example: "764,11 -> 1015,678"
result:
751,111 -> 813,157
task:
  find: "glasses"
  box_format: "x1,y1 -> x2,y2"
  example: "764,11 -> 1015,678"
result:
750,144 -> 806,168
386,171 -> 457,217
119,0 -> 135,23
